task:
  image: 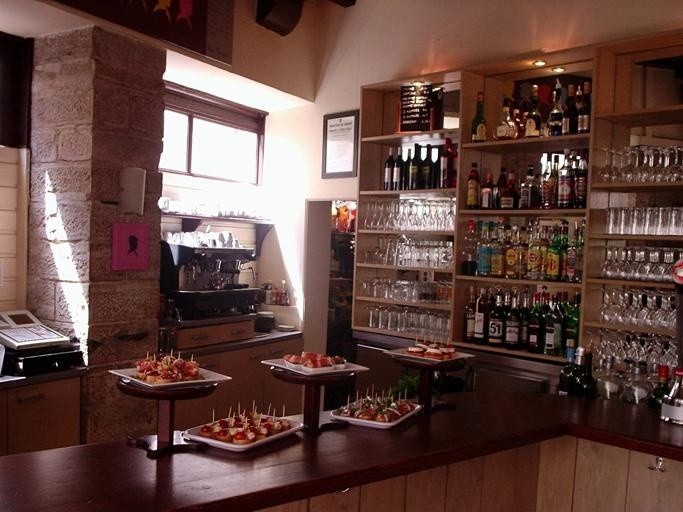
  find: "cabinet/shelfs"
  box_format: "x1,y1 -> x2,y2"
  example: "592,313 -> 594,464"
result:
0,367 -> 82,455
160,331 -> 301,431
325,199 -> 355,323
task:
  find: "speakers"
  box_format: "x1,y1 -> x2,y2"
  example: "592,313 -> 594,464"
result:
161,240 -> 179,291
255,0 -> 306,38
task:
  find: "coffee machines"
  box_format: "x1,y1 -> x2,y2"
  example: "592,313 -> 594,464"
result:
161,240 -> 259,323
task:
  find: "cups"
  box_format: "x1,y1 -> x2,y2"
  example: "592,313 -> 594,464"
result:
361,241 -> 454,339
164,228 -> 242,247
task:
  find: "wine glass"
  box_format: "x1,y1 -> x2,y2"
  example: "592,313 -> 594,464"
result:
363,200 -> 454,231
600,246 -> 675,334
597,143 -> 680,181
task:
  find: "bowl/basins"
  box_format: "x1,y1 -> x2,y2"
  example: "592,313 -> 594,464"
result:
463,81 -> 591,354
260,354 -> 368,378
604,205 -> 683,239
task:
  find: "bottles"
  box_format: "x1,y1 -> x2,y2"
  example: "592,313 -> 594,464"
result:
384,142 -> 457,189
259,278 -> 289,306
158,326 -> 176,354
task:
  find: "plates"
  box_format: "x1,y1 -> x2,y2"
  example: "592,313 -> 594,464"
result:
329,396 -> 420,431
187,411 -> 303,452
384,348 -> 473,365
556,337 -> 683,428
108,365 -> 230,390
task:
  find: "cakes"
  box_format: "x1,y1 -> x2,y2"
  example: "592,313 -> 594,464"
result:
135,356 -> 200,383
406,341 -> 456,360
340,398 -> 414,423
199,412 -> 290,445
